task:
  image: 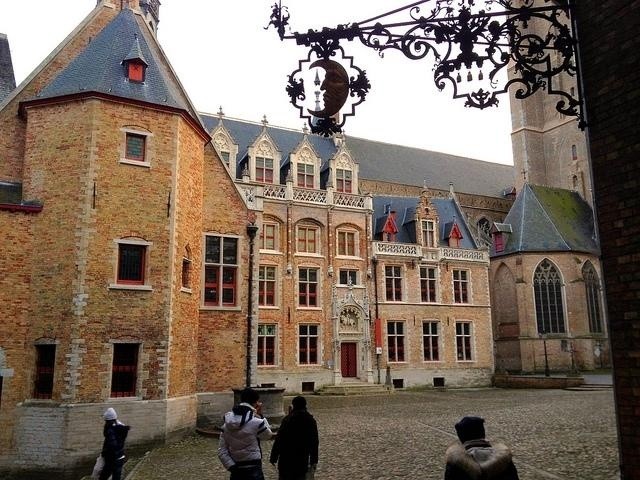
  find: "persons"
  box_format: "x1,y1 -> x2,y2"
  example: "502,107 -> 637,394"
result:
216,388 -> 273,480
443,417 -> 520,480
268,394 -> 320,480
95,407 -> 131,480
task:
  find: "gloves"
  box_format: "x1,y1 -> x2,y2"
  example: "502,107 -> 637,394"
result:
230,467 -> 242,477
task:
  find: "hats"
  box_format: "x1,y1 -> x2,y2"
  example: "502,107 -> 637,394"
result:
455,416 -> 485,443
292,397 -> 306,408
103,408 -> 117,420
240,388 -> 260,405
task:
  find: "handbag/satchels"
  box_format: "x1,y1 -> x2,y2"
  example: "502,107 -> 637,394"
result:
91,454 -> 105,476
306,465 -> 315,480
